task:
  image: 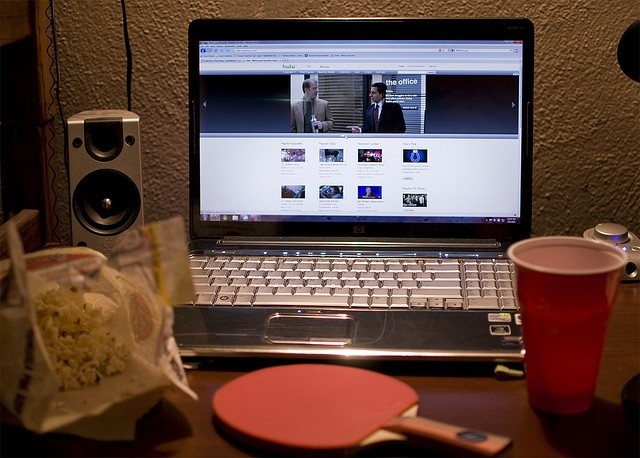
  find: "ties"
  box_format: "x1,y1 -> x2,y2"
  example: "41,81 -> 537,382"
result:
373,104 -> 379,133
304,102 -> 312,132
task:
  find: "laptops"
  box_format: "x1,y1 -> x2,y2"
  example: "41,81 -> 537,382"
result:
167,16 -> 535,364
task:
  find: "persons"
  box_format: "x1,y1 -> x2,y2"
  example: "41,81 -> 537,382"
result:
346,82 -> 407,133
405,194 -> 425,207
364,188 -> 375,197
291,79 -> 335,133
363,150 -> 375,163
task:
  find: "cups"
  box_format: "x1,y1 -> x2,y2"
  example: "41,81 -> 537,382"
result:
502,235 -> 630,416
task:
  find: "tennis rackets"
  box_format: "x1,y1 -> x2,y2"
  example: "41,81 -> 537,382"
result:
211,365 -> 512,457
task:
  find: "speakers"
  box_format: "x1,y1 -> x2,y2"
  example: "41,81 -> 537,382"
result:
584,220 -> 636,283
67,107 -> 144,250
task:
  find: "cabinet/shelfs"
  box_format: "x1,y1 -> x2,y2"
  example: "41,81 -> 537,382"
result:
1,1 -> 60,260
